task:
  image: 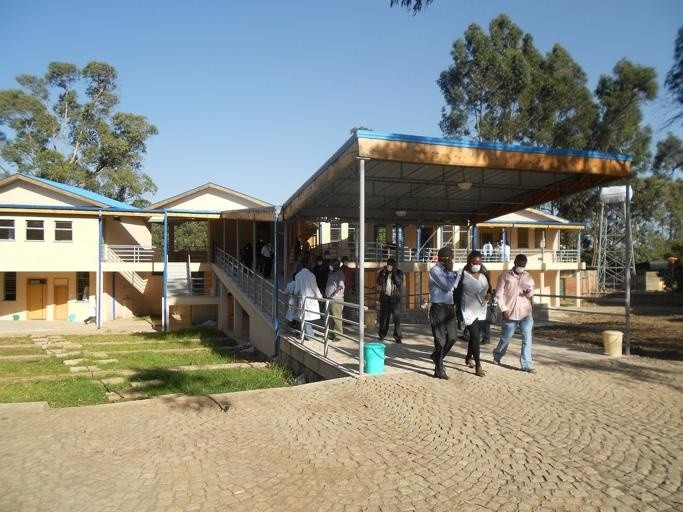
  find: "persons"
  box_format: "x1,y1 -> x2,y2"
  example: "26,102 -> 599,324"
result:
483,240 -> 511,262
377,258 -> 404,343
457,251 -> 493,377
494,255 -> 538,373
241,235 -> 353,341
429,248 -> 462,379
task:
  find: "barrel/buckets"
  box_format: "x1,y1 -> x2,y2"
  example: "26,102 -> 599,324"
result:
601,330 -> 624,357
364,343 -> 386,375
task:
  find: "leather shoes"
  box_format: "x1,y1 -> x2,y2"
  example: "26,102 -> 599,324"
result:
431,352 -> 448,380
330,336 -> 341,342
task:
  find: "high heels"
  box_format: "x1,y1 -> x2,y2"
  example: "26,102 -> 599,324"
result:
476,367 -> 485,376
466,361 -> 475,368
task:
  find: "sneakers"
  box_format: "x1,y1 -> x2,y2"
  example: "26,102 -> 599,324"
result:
523,368 -> 537,374
493,350 -> 500,364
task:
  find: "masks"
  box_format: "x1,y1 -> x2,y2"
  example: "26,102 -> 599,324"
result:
329,265 -> 333,270
387,265 -> 393,272
515,267 -> 525,273
471,263 -> 481,272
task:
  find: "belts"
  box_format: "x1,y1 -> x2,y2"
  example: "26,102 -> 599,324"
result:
434,303 -> 455,308
